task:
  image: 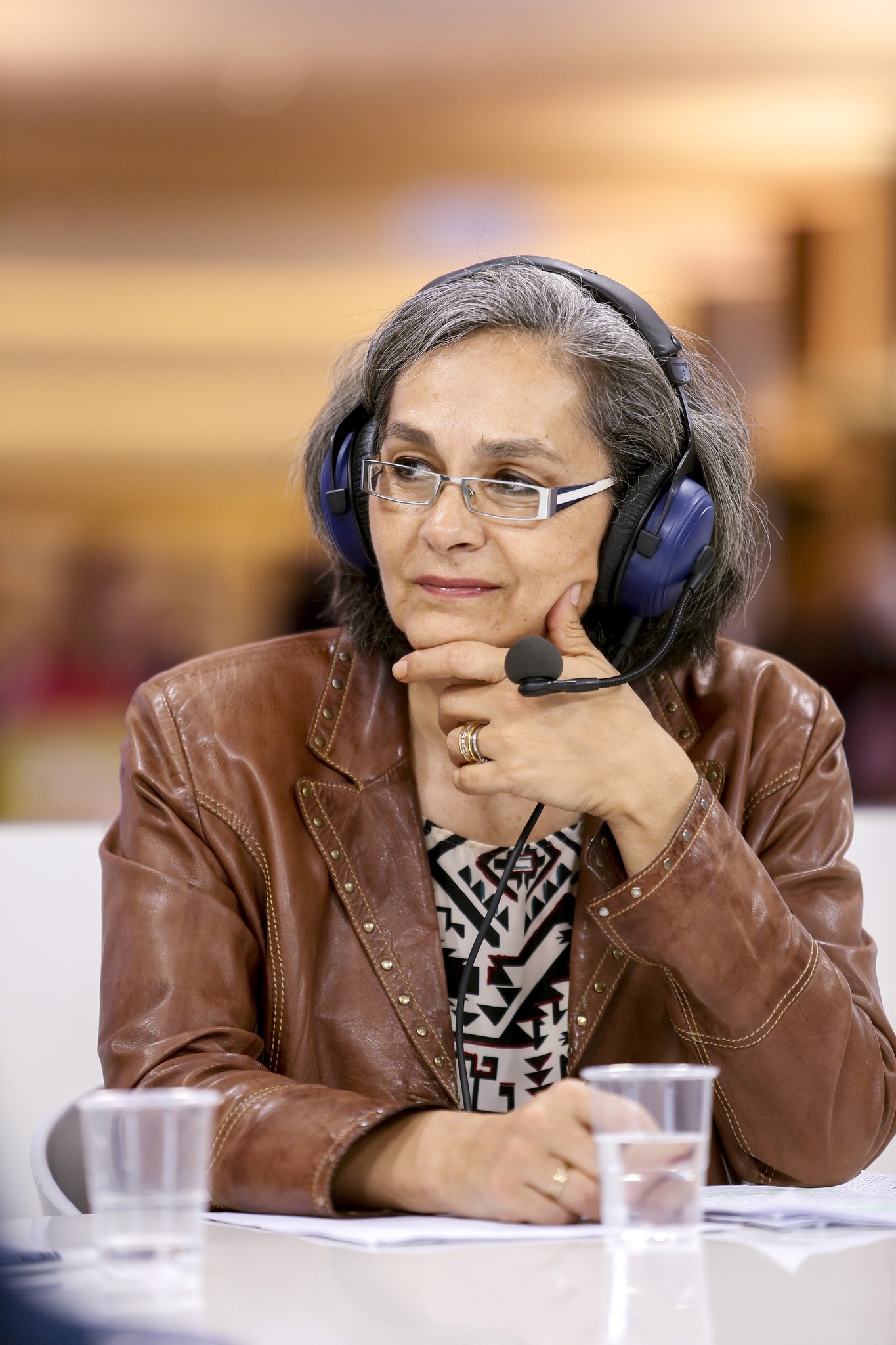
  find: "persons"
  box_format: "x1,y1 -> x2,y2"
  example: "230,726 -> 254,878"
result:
93,255 -> 896,1232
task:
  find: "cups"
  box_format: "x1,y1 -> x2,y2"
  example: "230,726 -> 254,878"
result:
80,1087 -> 220,1268
579,1061 -> 719,1233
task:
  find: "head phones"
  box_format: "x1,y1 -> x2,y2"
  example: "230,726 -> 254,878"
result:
317,254 -> 716,621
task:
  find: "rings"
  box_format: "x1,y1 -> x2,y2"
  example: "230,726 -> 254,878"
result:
458,722 -> 490,764
546,1162 -> 575,1201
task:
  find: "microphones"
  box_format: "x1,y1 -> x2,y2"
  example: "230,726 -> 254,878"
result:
504,543 -> 720,698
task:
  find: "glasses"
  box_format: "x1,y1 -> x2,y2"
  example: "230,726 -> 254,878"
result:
361,455 -> 620,522
